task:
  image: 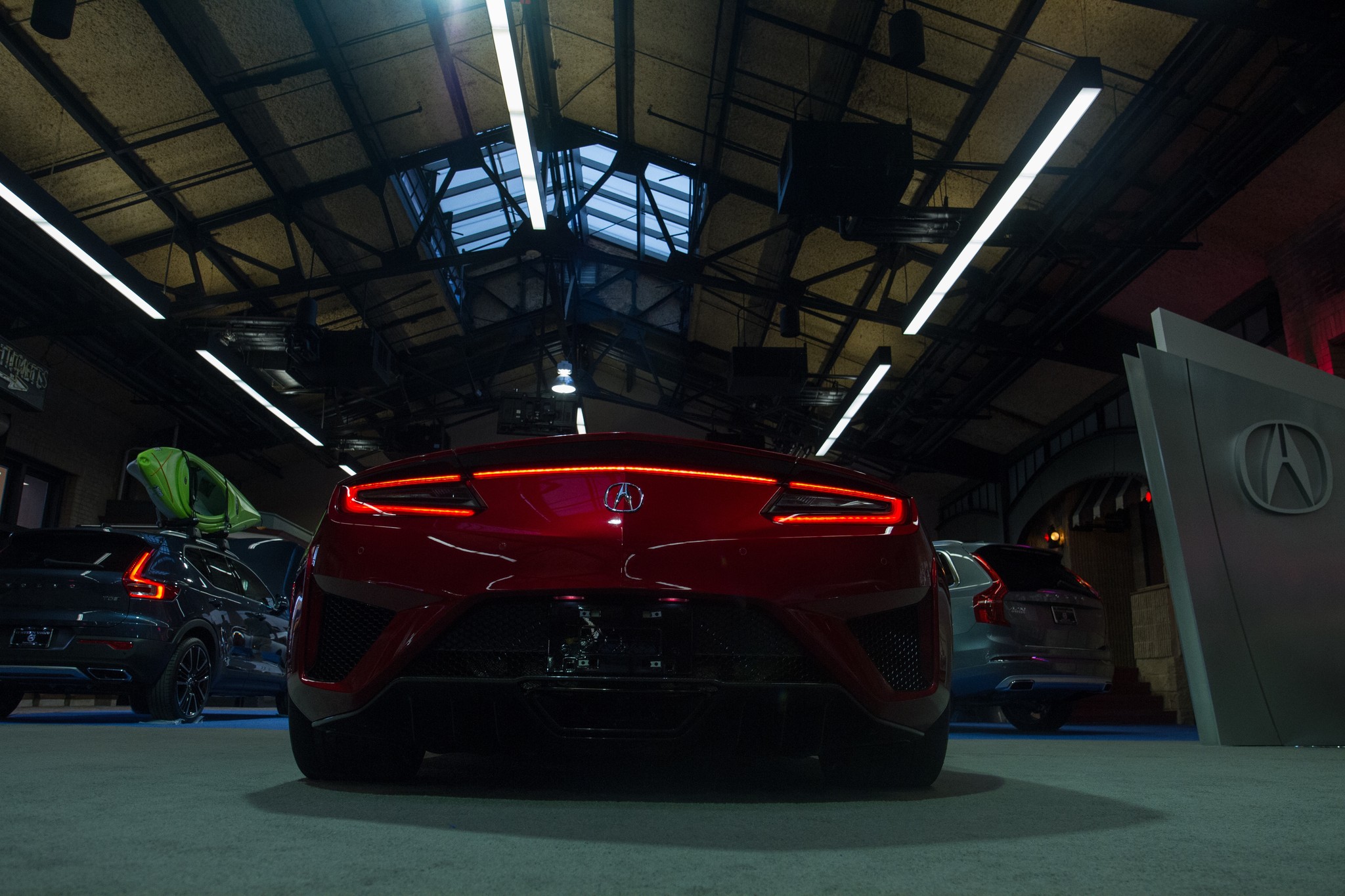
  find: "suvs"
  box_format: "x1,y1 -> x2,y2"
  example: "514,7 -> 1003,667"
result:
0,523 -> 287,724
934,539 -> 1112,733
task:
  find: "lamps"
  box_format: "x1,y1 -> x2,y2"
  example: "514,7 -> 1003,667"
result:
193,335 -> 326,449
295,246 -> 317,328
487,0 -> 548,231
900,54 -> 1105,337
816,345 -> 894,455
30,0 -> 77,39
337,451 -> 357,476
550,361 -> 577,397
889,0 -> 925,69
0,152 -> 178,319
779,305 -> 801,338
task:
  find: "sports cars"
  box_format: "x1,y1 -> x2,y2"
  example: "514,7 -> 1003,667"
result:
285,433 -> 952,790
